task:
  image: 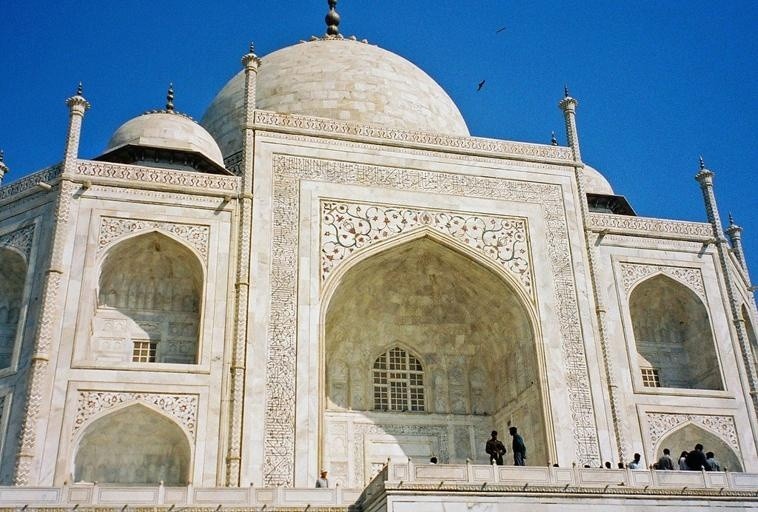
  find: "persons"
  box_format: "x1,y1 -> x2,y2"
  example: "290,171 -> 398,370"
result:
658,448 -> 676,470
684,443 -> 714,471
705,450 -> 722,472
677,449 -> 690,470
509,425 -> 527,466
628,453 -> 644,469
485,429 -> 507,465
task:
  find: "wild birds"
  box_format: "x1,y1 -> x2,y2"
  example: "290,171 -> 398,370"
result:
496,28 -> 506,32
477,80 -> 485,91
